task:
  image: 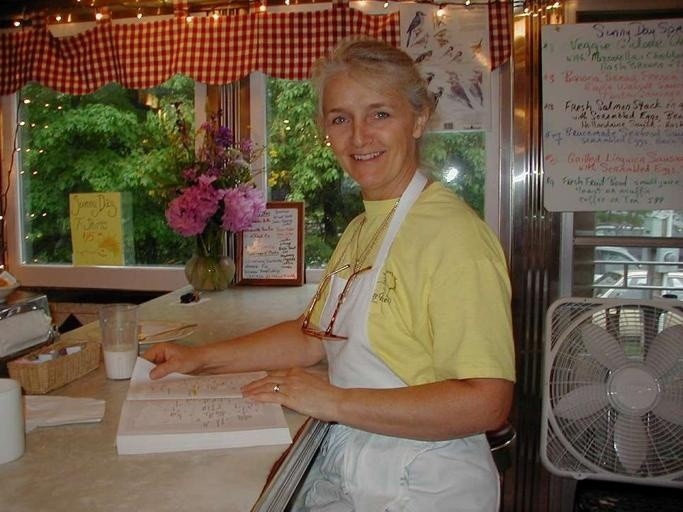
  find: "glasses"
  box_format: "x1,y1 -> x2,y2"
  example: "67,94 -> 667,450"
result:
302,265 -> 370,340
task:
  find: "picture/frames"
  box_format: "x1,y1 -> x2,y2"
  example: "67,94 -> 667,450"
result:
235,201 -> 305,287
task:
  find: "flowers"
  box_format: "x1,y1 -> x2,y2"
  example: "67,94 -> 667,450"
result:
164,101 -> 268,290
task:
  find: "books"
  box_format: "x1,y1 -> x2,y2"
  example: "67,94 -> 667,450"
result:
115,355 -> 294,455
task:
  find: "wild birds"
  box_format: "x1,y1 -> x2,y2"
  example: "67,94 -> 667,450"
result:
405,10 -> 484,109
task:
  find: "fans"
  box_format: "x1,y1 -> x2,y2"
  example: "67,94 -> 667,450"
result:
539,295 -> 682,488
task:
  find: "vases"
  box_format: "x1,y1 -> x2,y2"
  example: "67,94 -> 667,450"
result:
182,220 -> 235,290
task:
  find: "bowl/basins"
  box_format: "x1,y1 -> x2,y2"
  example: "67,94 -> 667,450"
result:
0,280 -> 20,302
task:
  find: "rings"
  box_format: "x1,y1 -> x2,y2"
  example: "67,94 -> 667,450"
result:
273,383 -> 280,394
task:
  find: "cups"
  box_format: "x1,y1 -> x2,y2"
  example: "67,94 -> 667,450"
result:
0,378 -> 27,464
98,303 -> 139,380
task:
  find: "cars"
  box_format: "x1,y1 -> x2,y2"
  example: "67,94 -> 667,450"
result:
592,224 -> 682,343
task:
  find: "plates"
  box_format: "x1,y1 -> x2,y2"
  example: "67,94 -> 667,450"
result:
93,318 -> 196,354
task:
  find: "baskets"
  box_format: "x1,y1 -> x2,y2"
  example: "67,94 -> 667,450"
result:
7,341 -> 100,393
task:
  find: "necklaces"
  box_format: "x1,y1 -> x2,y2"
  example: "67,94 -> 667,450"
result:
350,201 -> 398,275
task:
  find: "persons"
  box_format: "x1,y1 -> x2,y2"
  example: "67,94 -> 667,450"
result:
138,31 -> 518,512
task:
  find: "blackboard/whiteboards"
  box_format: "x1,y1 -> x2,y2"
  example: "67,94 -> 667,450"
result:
541,19 -> 683,215
